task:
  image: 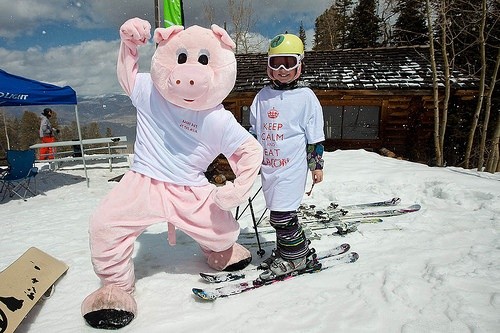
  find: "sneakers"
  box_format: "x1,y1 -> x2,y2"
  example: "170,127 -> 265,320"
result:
270,255 -> 306,276
260,250 -> 280,268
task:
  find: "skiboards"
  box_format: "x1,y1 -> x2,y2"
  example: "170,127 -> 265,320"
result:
253,196 -> 423,228
229,218 -> 385,248
191,241 -> 359,302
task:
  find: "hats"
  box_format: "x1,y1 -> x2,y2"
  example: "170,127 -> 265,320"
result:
44,108 -> 50,114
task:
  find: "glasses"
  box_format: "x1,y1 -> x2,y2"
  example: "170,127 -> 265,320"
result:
268,55 -> 299,71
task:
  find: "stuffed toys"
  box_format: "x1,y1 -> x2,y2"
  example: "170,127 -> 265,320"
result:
82,16 -> 263,330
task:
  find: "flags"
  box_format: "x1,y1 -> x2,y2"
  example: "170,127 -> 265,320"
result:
163,1 -> 185,30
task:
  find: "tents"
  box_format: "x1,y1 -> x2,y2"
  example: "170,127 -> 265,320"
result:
0,68 -> 88,182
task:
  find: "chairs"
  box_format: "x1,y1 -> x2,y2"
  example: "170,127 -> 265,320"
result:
0,150 -> 38,204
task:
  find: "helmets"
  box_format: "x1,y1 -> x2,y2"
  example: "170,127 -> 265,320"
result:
268,34 -> 304,55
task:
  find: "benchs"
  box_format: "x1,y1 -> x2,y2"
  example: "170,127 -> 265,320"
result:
30,136 -> 129,175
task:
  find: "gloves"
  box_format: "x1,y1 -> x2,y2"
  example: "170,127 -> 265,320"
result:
56,129 -> 60,133
48,130 -> 52,134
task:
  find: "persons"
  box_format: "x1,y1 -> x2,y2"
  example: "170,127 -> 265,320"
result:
249,34 -> 325,280
38,108 -> 57,160
